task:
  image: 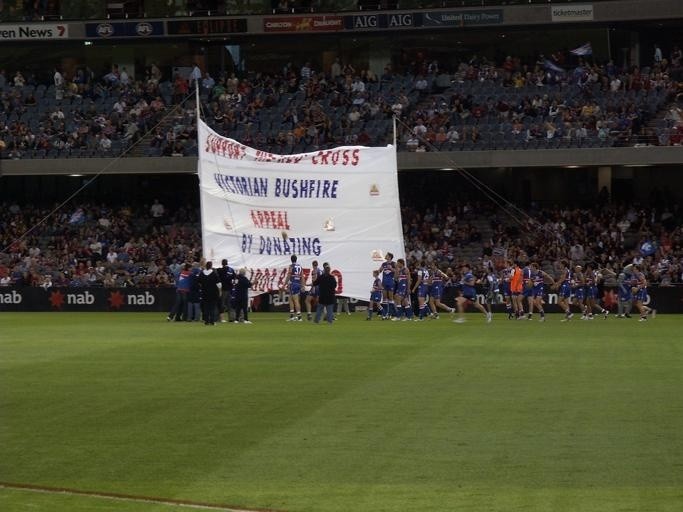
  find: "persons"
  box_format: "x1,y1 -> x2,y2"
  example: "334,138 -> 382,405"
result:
1,0 -> 683,161
1,176 -> 681,325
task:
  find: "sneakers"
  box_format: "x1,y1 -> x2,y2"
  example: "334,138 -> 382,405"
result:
450,307 -> 456,318
603,310 -> 610,320
284,315 -> 303,322
306,313 -> 337,325
452,318 -> 464,323
486,312 -> 492,322
366,317 -> 372,320
614,313 -> 633,318
507,315 -> 533,320
560,313 -> 574,322
166,317 -> 252,326
377,312 -> 439,322
580,315 -> 594,320
538,317 -> 545,322
637,309 -> 657,322
